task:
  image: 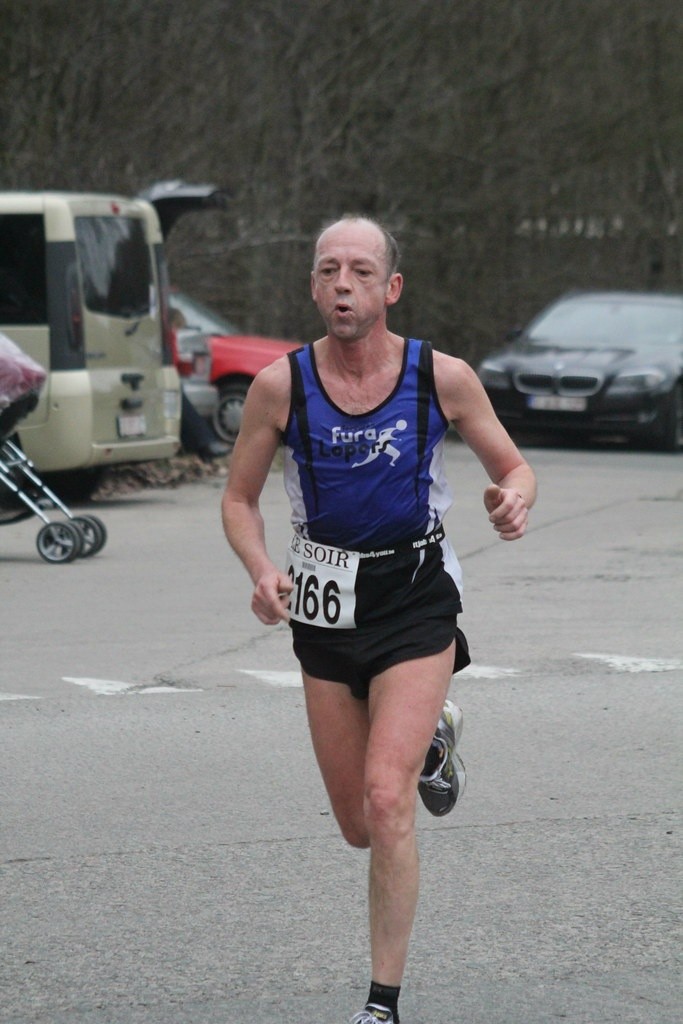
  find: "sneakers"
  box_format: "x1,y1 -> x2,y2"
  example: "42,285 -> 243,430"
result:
350,1003 -> 400,1024
419,700 -> 467,817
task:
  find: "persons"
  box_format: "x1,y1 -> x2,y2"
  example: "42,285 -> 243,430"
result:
221,212 -> 540,1024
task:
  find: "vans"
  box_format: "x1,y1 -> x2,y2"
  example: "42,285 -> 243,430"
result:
0,177 -> 216,508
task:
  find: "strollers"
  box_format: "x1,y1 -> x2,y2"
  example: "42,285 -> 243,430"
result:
0,337 -> 108,564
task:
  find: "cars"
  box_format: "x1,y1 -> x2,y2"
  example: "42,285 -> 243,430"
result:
474,286 -> 683,455
134,184 -> 306,447
